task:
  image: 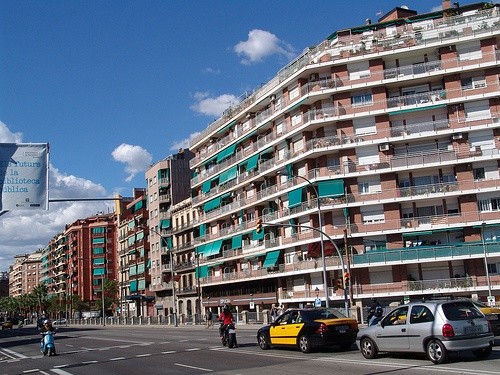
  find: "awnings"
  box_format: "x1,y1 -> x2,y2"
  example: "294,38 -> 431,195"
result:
42,237 -> 73,283
288,187 -> 303,207
202,143 -> 259,211
135,201 -> 143,210
232,234 -> 242,249
129,220 -> 146,293
195,266 -> 211,278
263,250 -> 281,267
253,227 -> 264,240
159,220 -> 172,248
317,179 -> 345,198
93,227 -> 105,275
195,239 -> 224,258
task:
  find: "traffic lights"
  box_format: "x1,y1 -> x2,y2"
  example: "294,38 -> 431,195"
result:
256,219 -> 263,233
336,276 -> 343,289
343,273 -> 349,290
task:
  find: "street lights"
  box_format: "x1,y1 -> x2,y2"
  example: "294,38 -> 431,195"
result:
481,222 -> 494,307
314,287 -> 319,308
77,257 -> 105,327
276,171 -> 329,317
137,226 -> 178,327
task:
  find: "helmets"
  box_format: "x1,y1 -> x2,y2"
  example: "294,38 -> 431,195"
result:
44,320 -> 49,326
371,298 -> 377,301
223,305 -> 229,309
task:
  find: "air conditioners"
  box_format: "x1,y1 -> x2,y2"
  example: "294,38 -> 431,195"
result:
453,132 -> 463,140
379,143 -> 390,152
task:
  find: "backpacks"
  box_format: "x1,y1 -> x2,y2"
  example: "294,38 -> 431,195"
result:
375,303 -> 383,317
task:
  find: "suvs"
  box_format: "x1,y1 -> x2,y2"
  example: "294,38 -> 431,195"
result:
356,294 -> 494,364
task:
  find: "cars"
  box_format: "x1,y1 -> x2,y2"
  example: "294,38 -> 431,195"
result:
391,297 -> 500,335
257,308 -> 359,353
52,318 -> 66,325
2,321 -> 12,330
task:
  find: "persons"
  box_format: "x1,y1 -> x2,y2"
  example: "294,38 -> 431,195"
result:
271,304 -> 285,322
394,319 -> 406,325
205,310 -> 212,328
218,305 -> 235,340
367,298 -> 380,327
40,319 -> 56,348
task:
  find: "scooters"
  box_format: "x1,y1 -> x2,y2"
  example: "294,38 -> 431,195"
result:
37,326 -> 58,357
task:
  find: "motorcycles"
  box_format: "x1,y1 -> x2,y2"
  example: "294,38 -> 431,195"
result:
216,315 -> 236,348
